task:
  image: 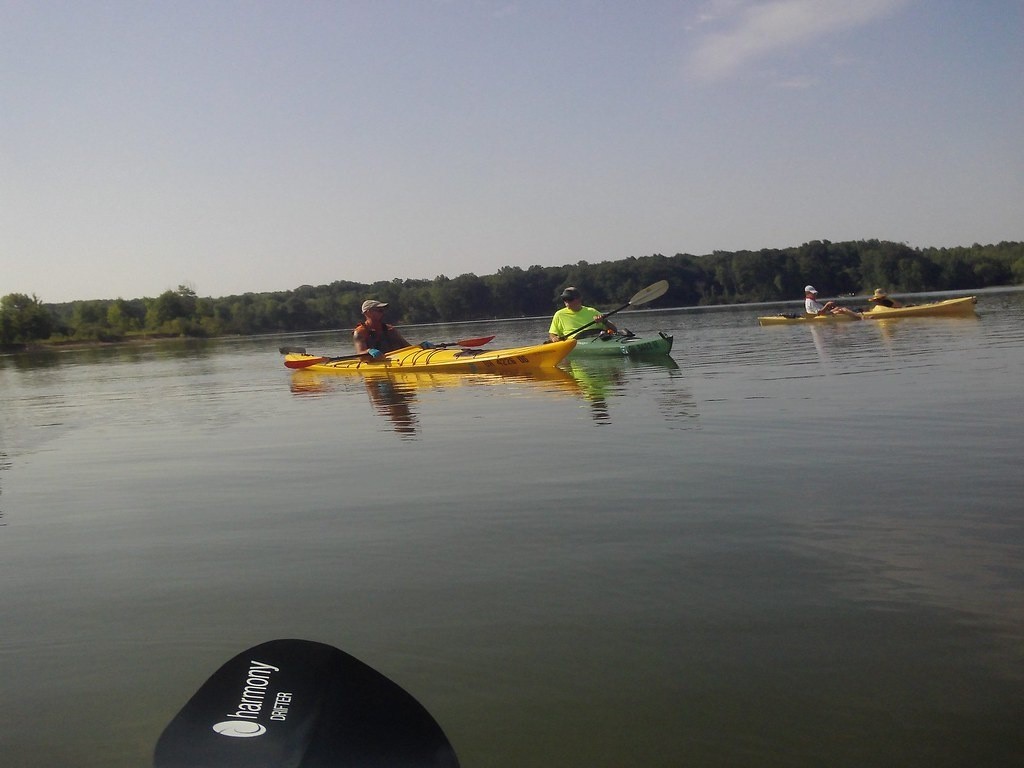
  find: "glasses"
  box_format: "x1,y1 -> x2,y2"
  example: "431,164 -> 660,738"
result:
371,308 -> 384,313
563,297 -> 578,301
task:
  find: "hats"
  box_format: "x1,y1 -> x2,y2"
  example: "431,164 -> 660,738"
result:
361,300 -> 389,313
872,288 -> 887,299
561,287 -> 581,299
805,286 -> 818,294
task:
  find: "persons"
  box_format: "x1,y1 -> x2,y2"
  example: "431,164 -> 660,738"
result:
805,285 -> 903,314
548,286 -> 617,342
353,300 -> 436,359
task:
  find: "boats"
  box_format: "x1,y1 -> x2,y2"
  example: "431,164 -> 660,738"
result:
541,331 -> 678,359
756,307 -> 863,326
277,341 -> 579,375
857,295 -> 981,320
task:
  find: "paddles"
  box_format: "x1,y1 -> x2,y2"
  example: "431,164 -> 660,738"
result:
542,279 -> 670,345
282,334 -> 497,370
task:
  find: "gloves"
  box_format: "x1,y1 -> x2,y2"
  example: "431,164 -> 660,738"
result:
421,341 -> 436,349
368,349 -> 386,361
